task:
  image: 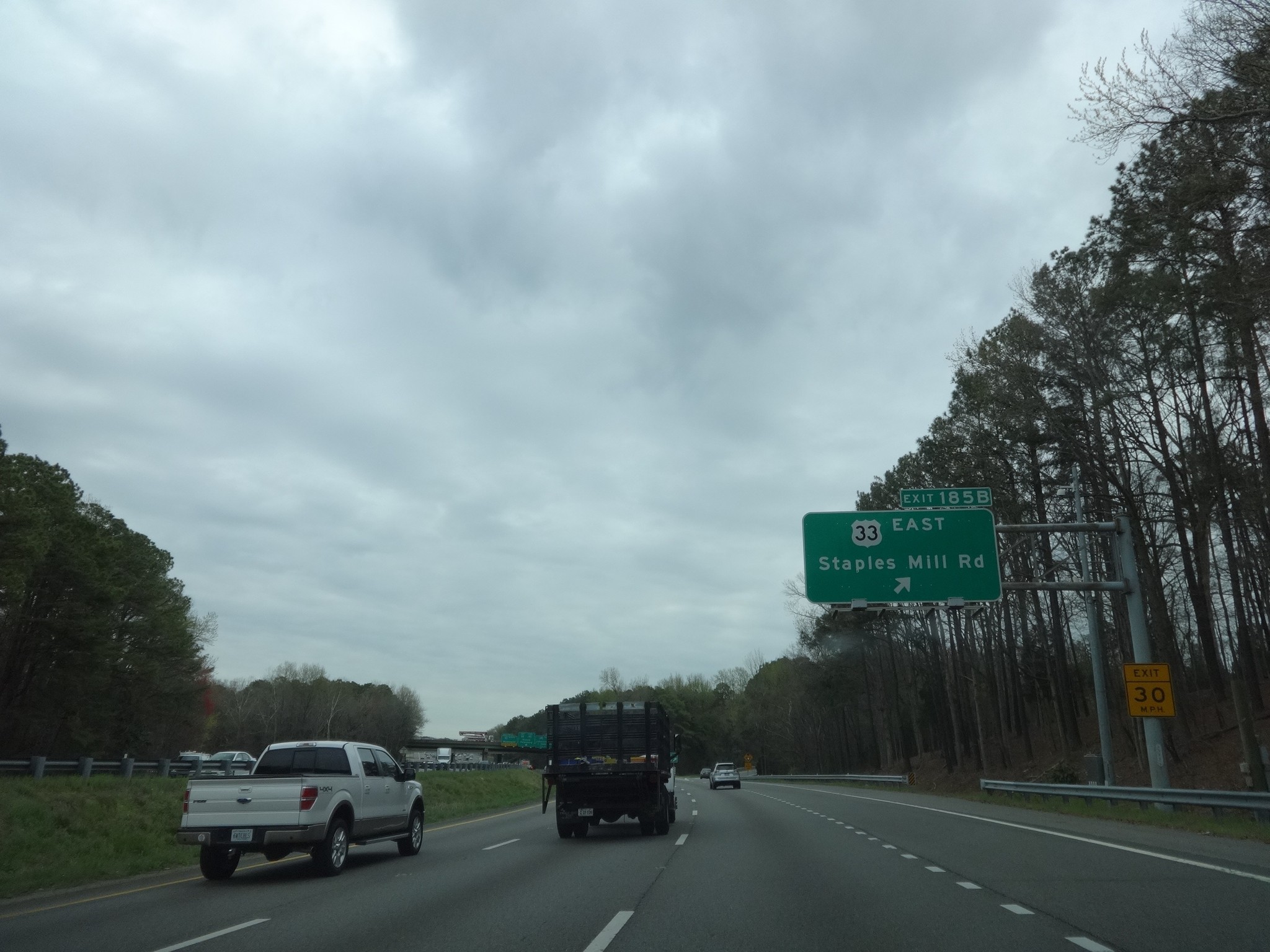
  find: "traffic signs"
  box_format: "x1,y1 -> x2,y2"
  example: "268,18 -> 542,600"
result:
535,735 -> 547,749
670,752 -> 678,763
800,507 -> 1002,607
501,735 -> 518,747
518,732 -> 536,747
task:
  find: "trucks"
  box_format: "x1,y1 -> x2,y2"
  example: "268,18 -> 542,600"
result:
436,748 -> 454,768
540,700 -> 683,840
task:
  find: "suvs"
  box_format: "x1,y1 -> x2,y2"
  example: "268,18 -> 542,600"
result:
708,762 -> 741,790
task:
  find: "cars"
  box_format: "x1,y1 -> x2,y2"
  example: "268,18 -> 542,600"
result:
699,768 -> 712,779
455,752 -> 474,764
420,751 -> 437,766
200,751 -> 257,779
478,760 -> 489,768
169,752 -> 210,777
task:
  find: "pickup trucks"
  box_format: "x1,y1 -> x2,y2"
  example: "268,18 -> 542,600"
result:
176,740 -> 427,880
